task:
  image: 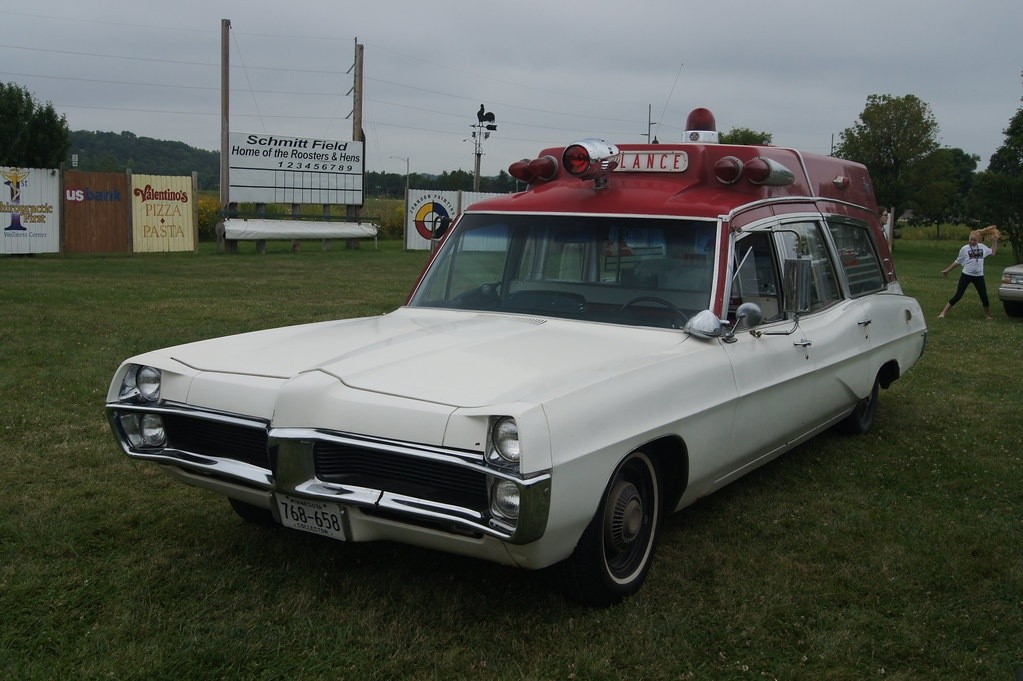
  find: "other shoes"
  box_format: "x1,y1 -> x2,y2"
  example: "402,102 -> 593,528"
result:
985,313 -> 993,320
937,315 -> 944,319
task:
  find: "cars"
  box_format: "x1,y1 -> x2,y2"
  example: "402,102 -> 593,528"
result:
105,108 -> 930,608
998,263 -> 1023,317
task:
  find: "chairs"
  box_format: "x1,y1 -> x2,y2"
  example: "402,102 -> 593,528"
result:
505,289 -> 590,315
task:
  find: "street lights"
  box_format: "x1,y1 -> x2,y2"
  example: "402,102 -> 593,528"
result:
390,156 -> 409,188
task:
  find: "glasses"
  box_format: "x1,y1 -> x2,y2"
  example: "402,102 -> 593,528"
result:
882,214 -> 888,217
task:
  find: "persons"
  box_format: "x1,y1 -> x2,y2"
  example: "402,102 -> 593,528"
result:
877,206 -> 891,252
936,225 -> 1000,321
